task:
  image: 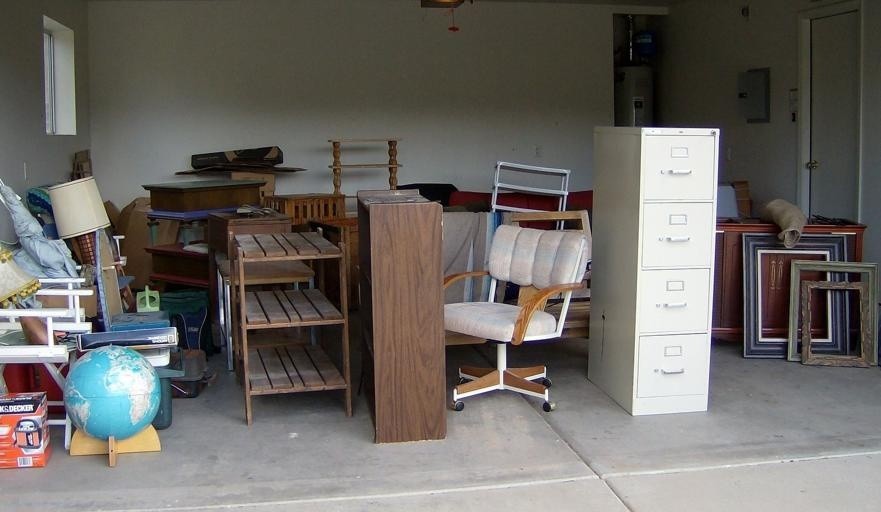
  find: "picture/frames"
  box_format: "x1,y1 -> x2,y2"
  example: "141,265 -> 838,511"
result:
741,231 -> 881,368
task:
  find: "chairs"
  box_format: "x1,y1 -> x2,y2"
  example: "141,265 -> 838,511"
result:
442,208 -> 594,412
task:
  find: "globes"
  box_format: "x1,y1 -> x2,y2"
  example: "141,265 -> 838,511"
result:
63,346 -> 162,442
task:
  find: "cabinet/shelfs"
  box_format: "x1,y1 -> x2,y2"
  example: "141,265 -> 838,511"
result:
712,217 -> 868,351
491,159 -> 574,231
588,124 -> 722,418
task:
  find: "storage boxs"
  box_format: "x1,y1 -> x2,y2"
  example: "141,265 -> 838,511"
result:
131,349 -> 173,429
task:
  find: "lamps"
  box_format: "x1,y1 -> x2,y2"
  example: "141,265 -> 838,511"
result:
1,250 -> 59,345
48,176 -> 111,268
